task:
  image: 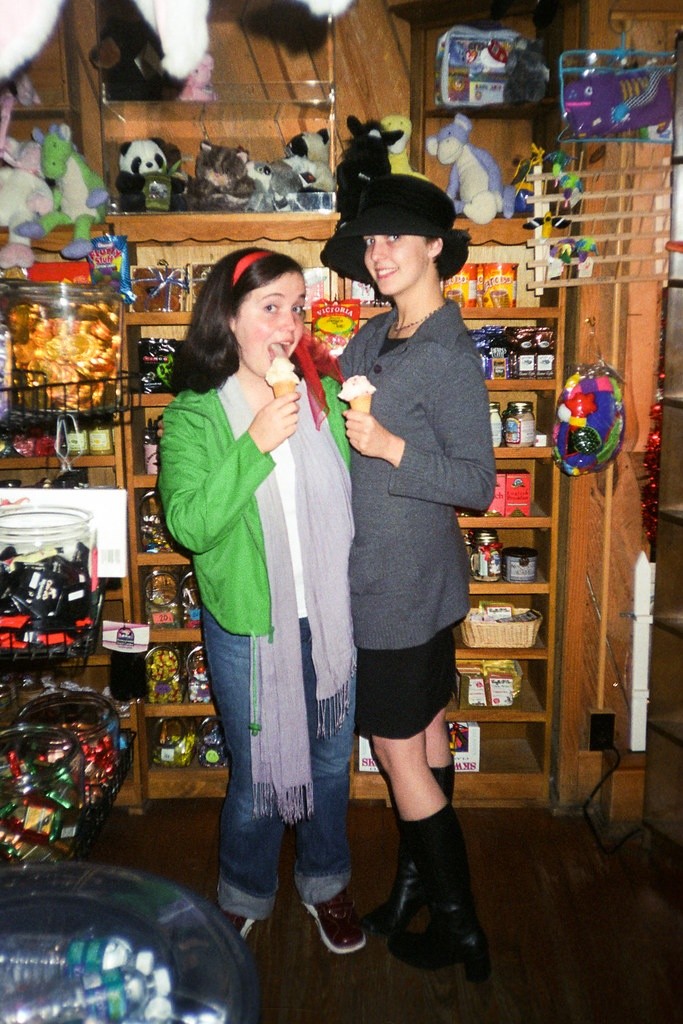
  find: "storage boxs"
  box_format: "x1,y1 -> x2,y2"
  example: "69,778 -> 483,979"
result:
447,721 -> 480,773
505,469 -> 531,517
482,469 -> 505,517
358,735 -> 382,772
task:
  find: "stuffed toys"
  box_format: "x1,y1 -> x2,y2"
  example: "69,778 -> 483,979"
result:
0,0 -> 674,270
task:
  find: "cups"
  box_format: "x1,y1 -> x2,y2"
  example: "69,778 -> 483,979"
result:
0,505 -> 99,655
0,276 -> 124,415
8,690 -> 127,810
0,725 -> 88,866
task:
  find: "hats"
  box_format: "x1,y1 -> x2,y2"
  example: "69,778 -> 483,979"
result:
319,174 -> 471,287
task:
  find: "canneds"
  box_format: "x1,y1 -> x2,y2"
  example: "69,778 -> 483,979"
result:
502,546 -> 537,585
442,262 -> 520,308
64,421 -> 115,456
489,401 -> 536,448
463,529 -> 502,581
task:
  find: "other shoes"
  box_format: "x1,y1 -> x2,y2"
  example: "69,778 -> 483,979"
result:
219,907 -> 254,941
303,884 -> 368,954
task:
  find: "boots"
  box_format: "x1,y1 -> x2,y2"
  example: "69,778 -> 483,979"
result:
360,752 -> 490,983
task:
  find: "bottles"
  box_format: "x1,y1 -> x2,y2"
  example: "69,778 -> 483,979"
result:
488,403 -> 502,447
503,402 -> 536,448
462,528 -> 538,583
440,262 -> 518,309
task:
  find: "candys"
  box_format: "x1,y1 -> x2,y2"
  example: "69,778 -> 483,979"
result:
142,512 -> 230,769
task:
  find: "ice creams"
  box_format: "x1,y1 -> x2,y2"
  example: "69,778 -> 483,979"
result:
338,375 -> 377,415
265,358 -> 299,398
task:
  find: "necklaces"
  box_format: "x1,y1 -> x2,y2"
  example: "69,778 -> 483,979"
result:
393,304 -> 442,331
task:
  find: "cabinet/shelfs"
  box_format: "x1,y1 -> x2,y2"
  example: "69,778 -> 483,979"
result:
0,250 -> 567,811
0,4 -> 76,189
94,0 -> 334,214
384,0 -> 553,218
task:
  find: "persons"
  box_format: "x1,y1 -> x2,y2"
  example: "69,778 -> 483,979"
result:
317,173 -> 499,972
156,245 -> 369,956
